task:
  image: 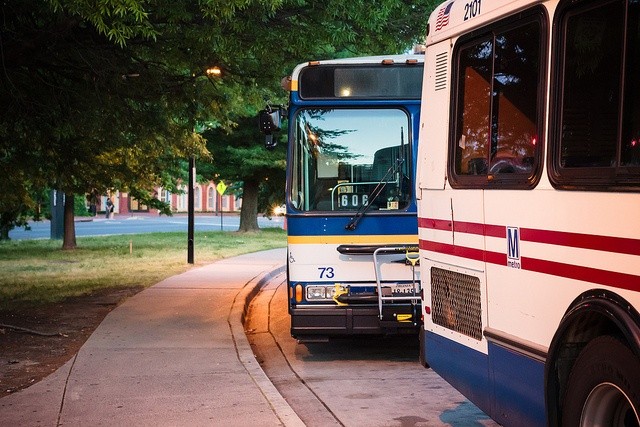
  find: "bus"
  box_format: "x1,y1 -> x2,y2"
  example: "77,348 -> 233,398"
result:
414,0 -> 639,427
257,43 -> 427,344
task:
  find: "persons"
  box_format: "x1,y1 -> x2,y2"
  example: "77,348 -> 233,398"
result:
104,196 -> 115,216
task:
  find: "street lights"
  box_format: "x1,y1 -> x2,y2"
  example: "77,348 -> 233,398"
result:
187,64 -> 223,265
